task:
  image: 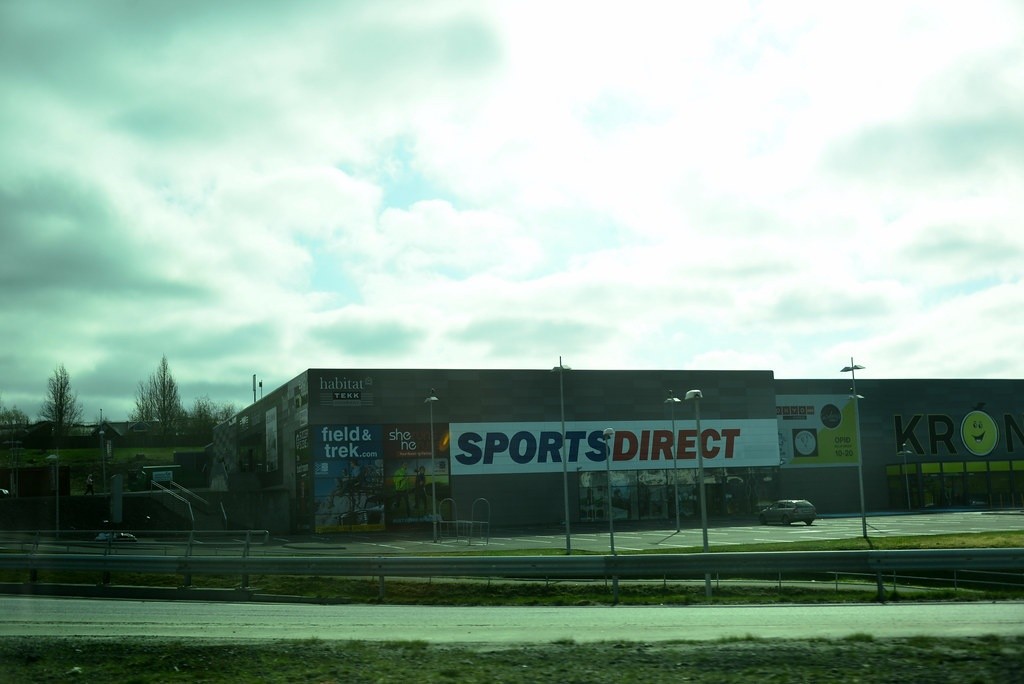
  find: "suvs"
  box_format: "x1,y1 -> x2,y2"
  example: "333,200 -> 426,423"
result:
758,501 -> 817,526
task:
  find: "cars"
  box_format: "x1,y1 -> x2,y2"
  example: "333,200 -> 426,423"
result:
96,533 -> 138,543
0,489 -> 9,497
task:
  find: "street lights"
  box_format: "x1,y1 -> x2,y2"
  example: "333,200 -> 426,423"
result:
99,431 -> 106,493
1,428 -> 29,496
684,390 -> 711,601
897,450 -> 912,510
551,367 -> 571,555
44,454 -> 59,540
841,365 -> 867,538
602,428 -> 616,551
425,397 -> 440,543
664,397 -> 682,532
